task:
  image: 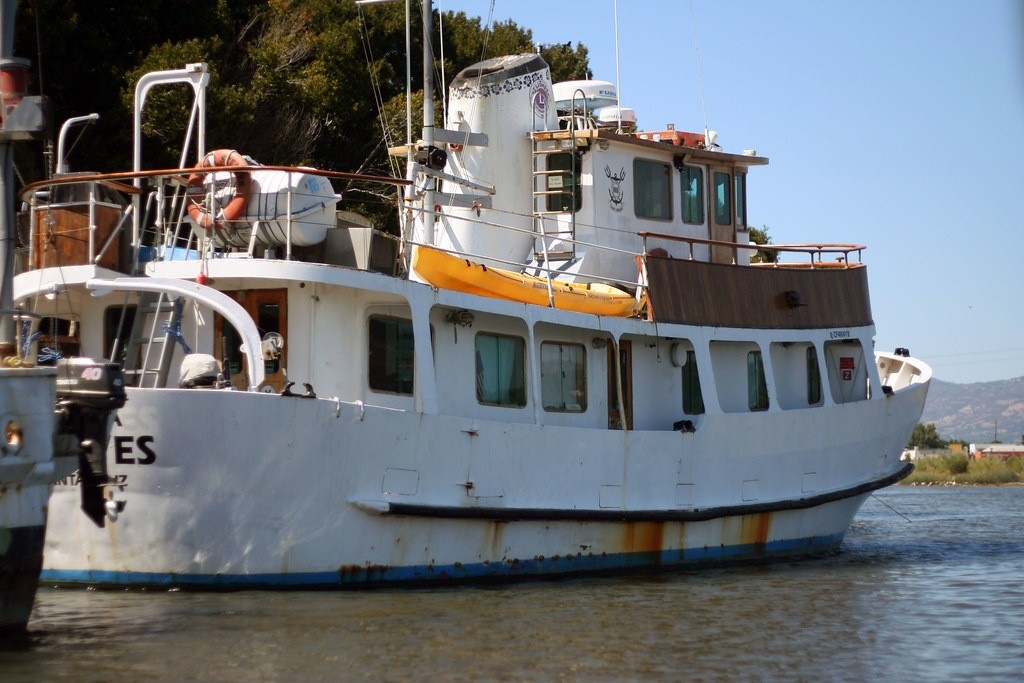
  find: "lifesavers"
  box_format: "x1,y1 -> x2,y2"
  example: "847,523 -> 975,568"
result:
183,147 -> 254,229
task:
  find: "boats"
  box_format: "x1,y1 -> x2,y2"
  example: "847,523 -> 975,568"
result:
0,0 -> 932,591
411,241 -> 634,317
